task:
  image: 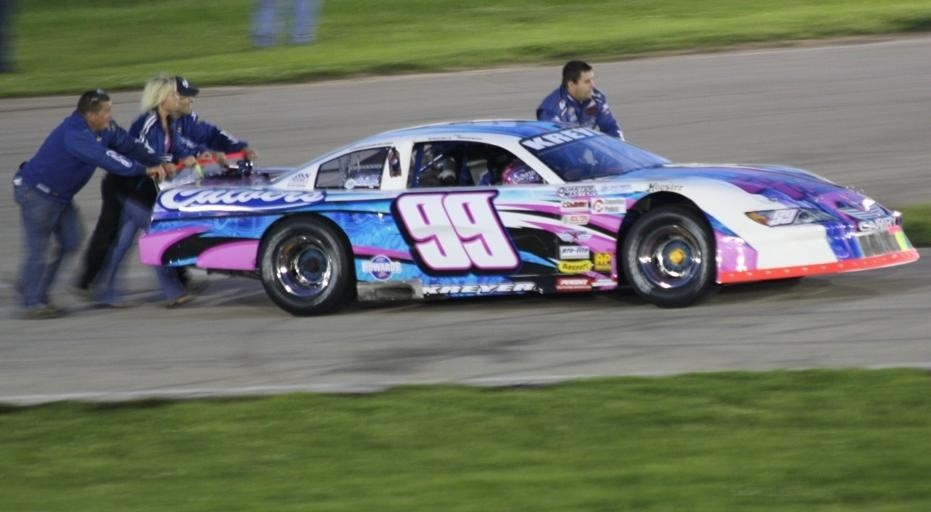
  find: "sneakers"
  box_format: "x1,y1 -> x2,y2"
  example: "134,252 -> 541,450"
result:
19,301 -> 64,320
67,276 -> 208,314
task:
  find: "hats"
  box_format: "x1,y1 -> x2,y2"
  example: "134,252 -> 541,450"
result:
176,77 -> 198,97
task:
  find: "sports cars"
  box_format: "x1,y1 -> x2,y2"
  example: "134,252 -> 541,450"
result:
131,115 -> 920,319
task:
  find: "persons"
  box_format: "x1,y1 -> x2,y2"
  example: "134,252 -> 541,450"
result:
534,57 -> 627,145
87,74 -> 213,311
11,87 -> 178,323
64,74 -> 260,302
249,1 -> 321,51
498,149 -> 573,184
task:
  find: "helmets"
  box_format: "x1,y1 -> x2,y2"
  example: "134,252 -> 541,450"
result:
498,159 -> 536,184
